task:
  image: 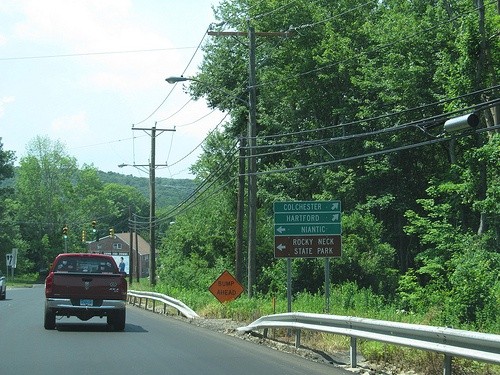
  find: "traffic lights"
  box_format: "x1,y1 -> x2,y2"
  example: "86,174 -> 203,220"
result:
81,230 -> 86,243
92,221 -> 96,230
109,228 -> 115,239
64,227 -> 68,239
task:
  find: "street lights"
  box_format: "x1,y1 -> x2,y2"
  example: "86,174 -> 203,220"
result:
166,75 -> 255,300
118,162 -> 159,290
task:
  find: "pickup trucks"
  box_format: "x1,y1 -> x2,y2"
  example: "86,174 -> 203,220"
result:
44,253 -> 128,331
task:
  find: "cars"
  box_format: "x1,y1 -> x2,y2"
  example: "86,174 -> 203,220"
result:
0,271 -> 7,300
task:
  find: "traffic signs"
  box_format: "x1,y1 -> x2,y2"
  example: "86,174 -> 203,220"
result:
273,212 -> 342,224
273,200 -> 342,212
275,235 -> 342,259
276,224 -> 343,235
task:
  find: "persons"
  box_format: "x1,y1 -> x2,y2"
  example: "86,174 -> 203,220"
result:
119,257 -> 125,272
